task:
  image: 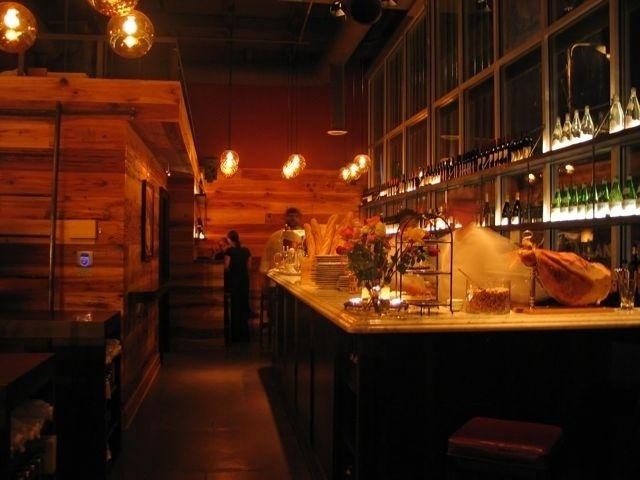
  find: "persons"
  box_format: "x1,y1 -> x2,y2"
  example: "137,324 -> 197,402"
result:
438,185 -> 544,306
215,236 -> 232,332
387,210 -> 434,291
261,206 -> 309,356
223,231 -> 252,347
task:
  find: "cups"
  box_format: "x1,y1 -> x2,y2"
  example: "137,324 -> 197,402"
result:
271,247 -> 307,274
465,279 -> 511,315
618,270 -> 636,310
447,299 -> 464,312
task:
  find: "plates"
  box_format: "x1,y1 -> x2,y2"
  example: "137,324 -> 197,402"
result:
309,256 -> 364,298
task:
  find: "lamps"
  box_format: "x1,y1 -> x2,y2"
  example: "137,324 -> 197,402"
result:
219,42 -> 371,184
0,0 -> 158,61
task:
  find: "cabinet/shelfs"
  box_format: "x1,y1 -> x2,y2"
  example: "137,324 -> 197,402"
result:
0,310 -> 123,480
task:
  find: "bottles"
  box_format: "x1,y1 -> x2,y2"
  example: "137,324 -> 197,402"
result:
362,170 -> 639,235
362,89 -> 639,206
